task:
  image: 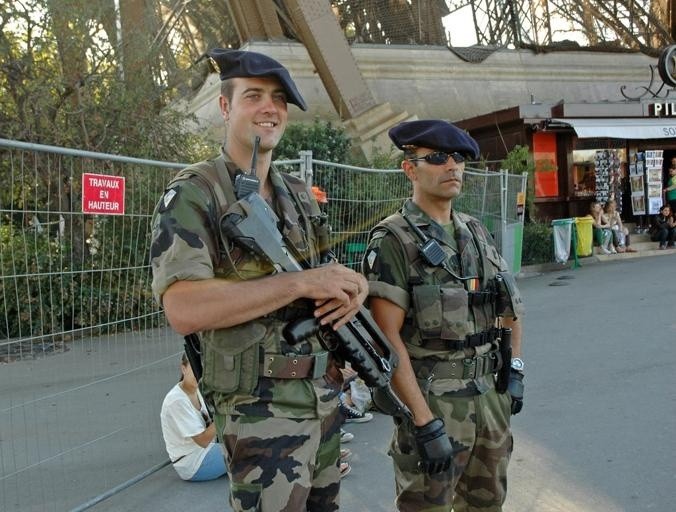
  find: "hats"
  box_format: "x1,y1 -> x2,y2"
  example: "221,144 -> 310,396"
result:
209,49 -> 307,110
389,120 -> 480,160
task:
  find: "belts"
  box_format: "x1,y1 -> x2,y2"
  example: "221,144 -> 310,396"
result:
410,351 -> 502,378
262,351 -> 334,379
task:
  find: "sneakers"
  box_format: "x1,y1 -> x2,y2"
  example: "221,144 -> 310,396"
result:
660,244 -> 675,249
340,403 -> 374,443
600,247 -> 636,254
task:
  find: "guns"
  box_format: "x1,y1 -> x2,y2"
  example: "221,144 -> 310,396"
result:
220,192 -> 415,419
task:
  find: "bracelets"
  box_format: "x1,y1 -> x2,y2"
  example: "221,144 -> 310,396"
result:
665,188 -> 668,192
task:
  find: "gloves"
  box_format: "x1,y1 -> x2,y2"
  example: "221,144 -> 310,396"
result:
508,371 -> 524,414
414,419 -> 453,474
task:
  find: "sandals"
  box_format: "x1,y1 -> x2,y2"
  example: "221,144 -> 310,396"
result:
340,448 -> 351,477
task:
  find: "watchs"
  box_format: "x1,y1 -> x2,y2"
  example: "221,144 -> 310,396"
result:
510,358 -> 525,373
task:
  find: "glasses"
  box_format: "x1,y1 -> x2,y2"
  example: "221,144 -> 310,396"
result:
408,151 -> 465,164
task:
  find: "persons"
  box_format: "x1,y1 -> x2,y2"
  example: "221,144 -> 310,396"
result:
604,200 -> 639,253
148,46 -> 369,512
587,203 -> 617,254
334,398 -> 373,442
160,353 -> 352,479
649,206 -> 676,249
671,157 -> 676,168
361,117 -> 526,512
661,169 -> 676,222
